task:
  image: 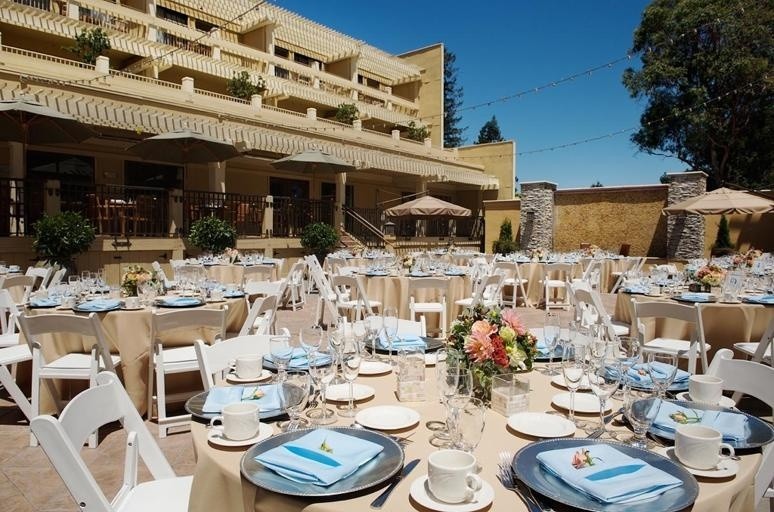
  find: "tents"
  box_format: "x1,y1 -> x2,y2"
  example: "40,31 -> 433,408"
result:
383,195 -> 471,239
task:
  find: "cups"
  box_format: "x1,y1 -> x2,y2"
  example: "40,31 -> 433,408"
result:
212,402 -> 262,439
426,449 -> 484,502
395,344 -> 426,401
225,354 -> 262,378
123,297 -> 141,309
689,375 -> 723,404
491,374 -> 529,418
674,425 -> 735,470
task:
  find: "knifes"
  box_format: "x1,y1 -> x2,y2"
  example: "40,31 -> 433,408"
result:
371,458 -> 421,509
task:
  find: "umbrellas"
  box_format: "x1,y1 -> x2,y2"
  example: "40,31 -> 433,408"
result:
269,145 -> 355,223
0,98 -> 96,178
660,186 -> 774,253
122,123 -> 244,235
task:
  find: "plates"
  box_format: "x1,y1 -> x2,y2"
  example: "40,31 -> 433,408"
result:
661,445 -> 739,479
409,471 -> 491,512
184,383 -> 309,422
552,391 -> 612,415
73,300 -> 119,310
239,427 -> 402,497
322,383 -> 374,402
364,335 -> 444,353
354,405 -> 419,431
627,395 -> 774,450
552,368 -> 605,391
510,439 -> 700,511
343,361 -> 393,376
223,293 -> 246,299
225,369 -> 273,381
206,424 -> 277,447
742,300 -> 774,305
674,391 -> 736,408
508,411 -> 577,438
117,307 -> 146,311
672,295 -> 718,302
155,299 -> 206,309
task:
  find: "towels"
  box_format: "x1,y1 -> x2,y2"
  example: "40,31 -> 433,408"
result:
535,442 -> 682,505
77,298 -> 122,311
263,347 -> 329,369
644,397 -> 752,449
682,294 -> 707,301
203,382 -> 284,417
31,298 -> 57,306
166,294 -> 199,307
749,292 -> 774,304
606,360 -> 691,388
379,328 -> 426,351
536,342 -> 573,356
253,428 -> 385,487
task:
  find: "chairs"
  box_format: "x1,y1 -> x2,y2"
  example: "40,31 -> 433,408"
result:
245,279 -> 287,335
24,266 -> 52,302
0,275 -> 34,307
223,295 -> 277,378
17,310 -> 123,449
29,370 -> 193,511
704,347 -> 774,512
194,328 -> 290,391
46,267 -> 67,290
538,263 -> 574,313
495,261 -> 529,309
565,279 -> 632,349
572,259 -> 605,306
304,254 -> 338,328
407,294 -> 446,341
279,261 -> 307,312
611,256 -> 647,295
0,288 -> 33,423
329,273 -> 381,321
731,310 -> 774,413
240,265 -> 274,291
147,304 -> 228,438
629,298 -> 711,379
151,261 -> 175,290
454,273 -> 505,308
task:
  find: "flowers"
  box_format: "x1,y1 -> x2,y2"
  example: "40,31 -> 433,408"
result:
732,249 -> 763,269
526,238 -> 545,262
401,251 -> 424,274
694,263 -> 726,291
224,247 -> 240,264
580,242 -> 598,258
444,302 -> 537,403
118,265 -> 159,299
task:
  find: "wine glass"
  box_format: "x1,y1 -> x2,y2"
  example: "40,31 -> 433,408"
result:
89,272 -> 98,300
338,339 -> 360,420
447,398 -> 487,456
384,306 -> 396,369
309,355 -> 339,426
585,357 -> 621,438
284,371 -> 313,432
178,267 -> 188,299
299,325 -> 323,358
99,268 -> 105,299
364,313 -> 384,362
436,348 -> 460,407
81,270 -> 91,300
545,311 -> 559,375
569,320 -> 587,370
441,367 -> 477,425
649,268 -> 687,293
586,325 -> 608,354
68,273 -> 80,295
328,317 -> 344,353
562,343 -> 584,427
588,341 -> 619,358
270,338 -> 293,382
627,383 -> 659,449
650,352 -> 679,398
612,335 -> 640,376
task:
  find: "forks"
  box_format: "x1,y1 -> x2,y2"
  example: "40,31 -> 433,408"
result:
497,447 -> 541,512
